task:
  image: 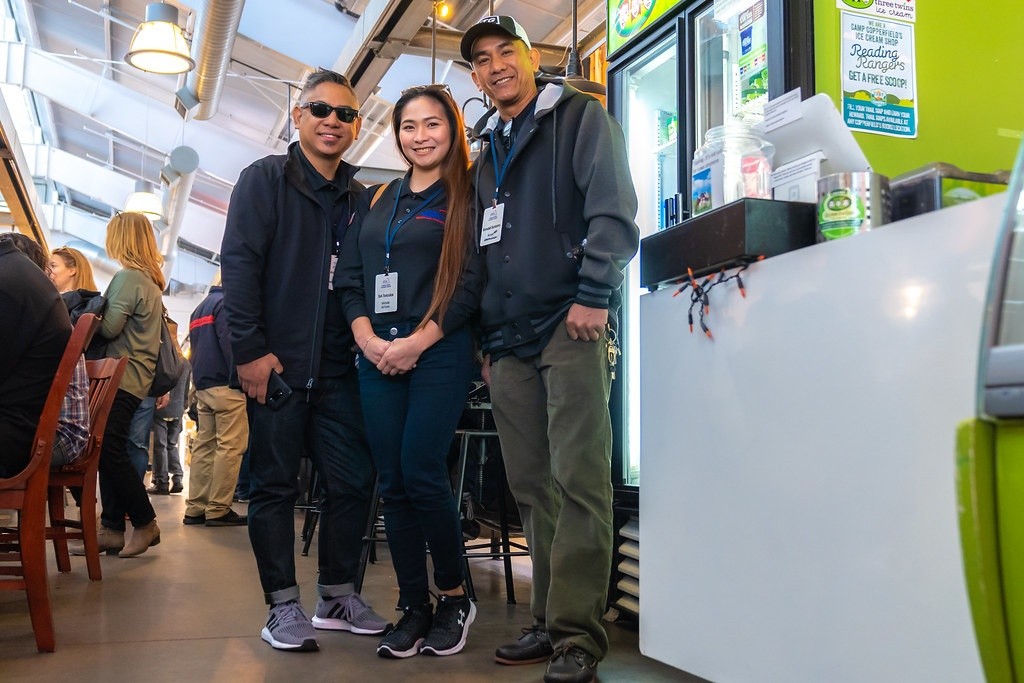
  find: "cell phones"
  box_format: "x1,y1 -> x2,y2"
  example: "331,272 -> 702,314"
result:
265,369 -> 293,412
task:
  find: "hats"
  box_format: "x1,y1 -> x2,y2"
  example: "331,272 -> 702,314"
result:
459,14 -> 531,69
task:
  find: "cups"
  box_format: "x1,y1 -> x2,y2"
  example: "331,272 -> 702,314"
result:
740,26 -> 752,56
666,116 -> 677,141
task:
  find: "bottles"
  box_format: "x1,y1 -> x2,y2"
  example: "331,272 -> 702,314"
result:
691,123 -> 775,218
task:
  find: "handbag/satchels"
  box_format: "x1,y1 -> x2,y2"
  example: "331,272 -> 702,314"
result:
148,315 -> 185,397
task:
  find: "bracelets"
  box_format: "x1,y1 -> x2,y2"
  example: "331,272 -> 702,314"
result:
362,335 -> 376,354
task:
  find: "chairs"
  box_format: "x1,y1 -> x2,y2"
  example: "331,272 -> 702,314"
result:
0,312 -> 103,653
45,354 -> 128,582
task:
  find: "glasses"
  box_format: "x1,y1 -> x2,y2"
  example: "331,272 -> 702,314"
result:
301,102 -> 359,123
116,210 -> 123,218
401,84 -> 453,99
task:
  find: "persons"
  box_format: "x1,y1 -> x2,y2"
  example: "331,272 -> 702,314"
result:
183,266 -> 248,526
146,316 -> 191,496
46,249 -> 107,364
339,84 -> 480,659
219,66 -> 394,653
459,14 -> 641,683
0,233 -> 72,482
68,211 -> 167,559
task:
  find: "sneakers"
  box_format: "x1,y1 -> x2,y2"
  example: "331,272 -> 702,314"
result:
376,602 -> 433,658
311,593 -> 393,634
259,599 -> 319,650
419,590 -> 478,656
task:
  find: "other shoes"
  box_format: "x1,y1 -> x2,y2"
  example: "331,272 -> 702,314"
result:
118,519 -> 161,557
183,514 -> 205,524
147,484 -> 169,495
67,525 -> 125,555
204,507 -> 248,525
170,483 -> 183,493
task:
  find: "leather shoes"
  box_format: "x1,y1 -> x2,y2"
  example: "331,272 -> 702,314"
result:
542,642 -> 596,683
495,625 -> 553,665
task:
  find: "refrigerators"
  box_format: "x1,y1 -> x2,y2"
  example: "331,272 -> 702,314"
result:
604,0 -> 1023,630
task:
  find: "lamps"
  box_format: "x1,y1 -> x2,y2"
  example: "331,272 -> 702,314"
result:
123,146 -> 164,221
550,0 -> 608,101
123,0 -> 197,75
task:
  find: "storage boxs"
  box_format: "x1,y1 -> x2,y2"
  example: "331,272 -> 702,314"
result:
637,196 -> 817,288
